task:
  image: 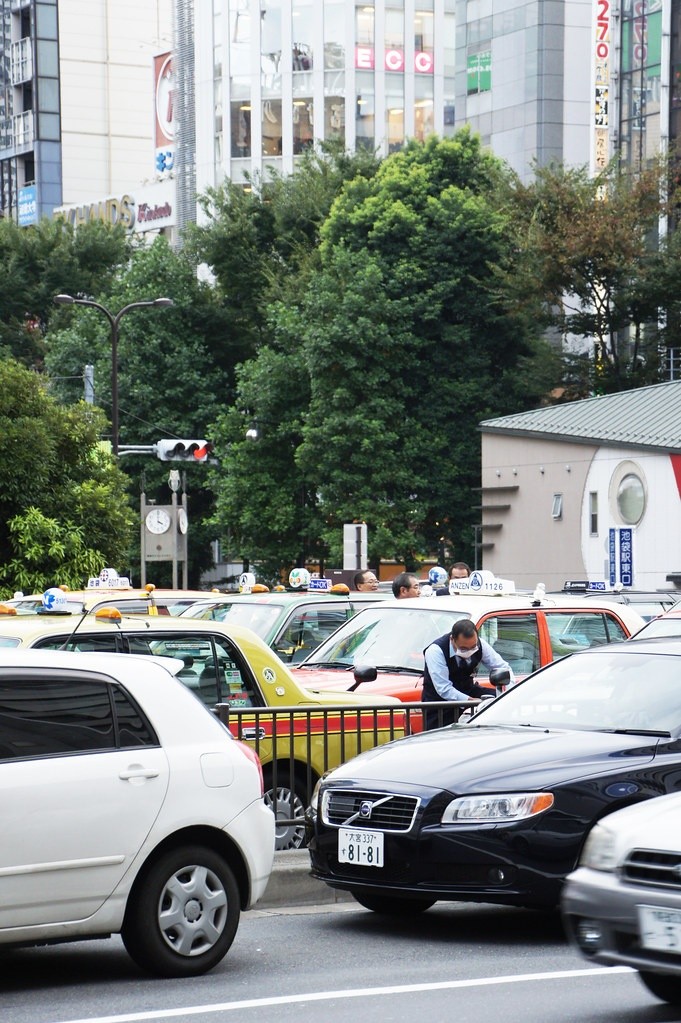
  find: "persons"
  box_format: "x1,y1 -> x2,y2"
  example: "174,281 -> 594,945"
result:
421,619 -> 516,730
392,572 -> 420,599
354,570 -> 378,592
436,561 -> 473,596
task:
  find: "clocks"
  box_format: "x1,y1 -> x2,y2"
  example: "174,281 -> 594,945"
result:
178,509 -> 188,534
146,509 -> 172,535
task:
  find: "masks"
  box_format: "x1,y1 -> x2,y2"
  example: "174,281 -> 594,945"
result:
453,639 -> 479,659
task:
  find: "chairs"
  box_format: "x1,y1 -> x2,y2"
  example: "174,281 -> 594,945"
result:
491,640 -> 534,676
173,653 -> 235,706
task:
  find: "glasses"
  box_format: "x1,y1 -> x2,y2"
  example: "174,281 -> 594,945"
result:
408,583 -> 422,589
361,580 -> 379,586
454,638 -> 478,653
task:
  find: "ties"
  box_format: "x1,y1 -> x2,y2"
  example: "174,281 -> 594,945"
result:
459,656 -> 467,668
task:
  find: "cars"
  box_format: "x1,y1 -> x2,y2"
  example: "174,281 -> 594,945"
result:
0,647 -> 277,979
0,608 -> 409,852
0,584 -> 229,618
303,636 -> 681,936
561,794 -> 681,1004
178,572 -> 396,669
295,567 -> 681,734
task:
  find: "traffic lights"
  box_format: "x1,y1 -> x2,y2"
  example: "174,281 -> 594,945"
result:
158,439 -> 212,462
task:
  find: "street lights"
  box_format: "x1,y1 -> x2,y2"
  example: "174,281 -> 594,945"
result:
54,293 -> 173,465
